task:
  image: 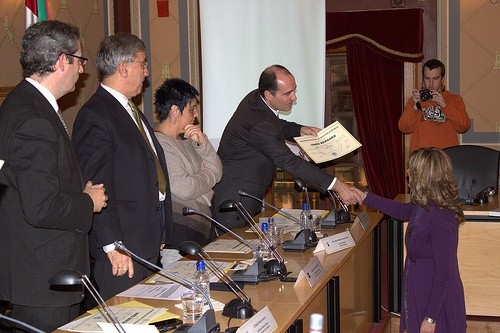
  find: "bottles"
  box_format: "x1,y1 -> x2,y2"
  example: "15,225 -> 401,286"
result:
300,204 -> 312,232
258,223 -> 272,259
192,261 -> 210,306
267,217 -> 278,247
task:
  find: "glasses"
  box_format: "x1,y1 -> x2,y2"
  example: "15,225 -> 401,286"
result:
131,60 -> 148,68
59,51 -> 88,65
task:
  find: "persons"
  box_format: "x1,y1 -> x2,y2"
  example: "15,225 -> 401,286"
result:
0,19 -> 109,332
210,64 -> 362,238
336,146 -> 468,333
398,59 -> 471,155
71,32 -> 172,303
152,78 -> 223,254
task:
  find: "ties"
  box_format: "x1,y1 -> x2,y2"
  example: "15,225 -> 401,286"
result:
128,98 -> 167,195
57,110 -> 71,141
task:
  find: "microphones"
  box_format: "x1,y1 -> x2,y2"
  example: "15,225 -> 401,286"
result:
114,241 -> 220,333
237,190 -> 311,250
181,206 -> 264,282
299,151 -> 339,227
465,178 -> 477,204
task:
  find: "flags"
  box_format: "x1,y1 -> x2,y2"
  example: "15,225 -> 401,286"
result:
24,0 -> 52,30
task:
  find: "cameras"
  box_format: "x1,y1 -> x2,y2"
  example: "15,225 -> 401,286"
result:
419,89 -> 432,101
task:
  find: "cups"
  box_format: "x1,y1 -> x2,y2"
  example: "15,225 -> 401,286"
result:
181,291 -> 204,324
253,240 -> 269,262
277,228 -> 283,243
312,214 -> 321,231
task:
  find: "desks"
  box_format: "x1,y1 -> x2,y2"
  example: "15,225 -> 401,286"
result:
392,194 -> 500,322
52,208 -> 382,333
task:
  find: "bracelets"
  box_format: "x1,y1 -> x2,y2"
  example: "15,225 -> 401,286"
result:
424,316 -> 435,324
